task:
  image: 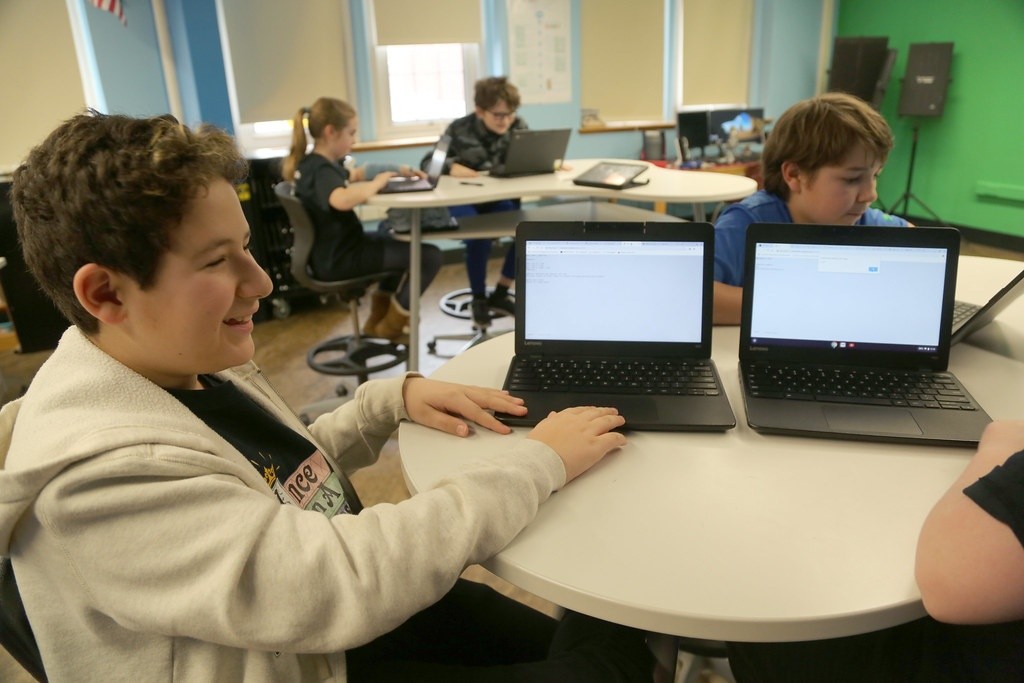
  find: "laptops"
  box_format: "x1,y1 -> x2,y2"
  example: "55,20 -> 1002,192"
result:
738,223 -> 997,447
495,221 -> 735,429
572,161 -> 649,190
950,269 -> 1024,345
377,134 -> 452,194
488,128 -> 571,177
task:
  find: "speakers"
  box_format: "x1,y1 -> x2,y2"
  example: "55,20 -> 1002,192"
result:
899,41 -> 954,116
828,35 -> 890,104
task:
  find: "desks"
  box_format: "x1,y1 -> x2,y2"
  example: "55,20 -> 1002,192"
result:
361,158 -> 758,372
350,119 -> 681,164
397,255 -> 1024,683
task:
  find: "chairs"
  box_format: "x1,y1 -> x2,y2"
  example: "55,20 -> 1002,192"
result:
418,151 -> 519,352
274,180 -> 408,386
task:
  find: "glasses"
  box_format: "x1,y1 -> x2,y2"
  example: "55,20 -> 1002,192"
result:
485,107 -> 517,118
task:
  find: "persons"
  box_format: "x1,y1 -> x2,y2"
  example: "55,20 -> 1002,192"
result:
1,113 -> 649,683
283,97 -> 443,346
713,92 -> 919,324
436,78 -> 572,332
725,418 -> 1024,683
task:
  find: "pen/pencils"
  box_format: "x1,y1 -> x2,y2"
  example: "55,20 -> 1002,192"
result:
460,181 -> 483,186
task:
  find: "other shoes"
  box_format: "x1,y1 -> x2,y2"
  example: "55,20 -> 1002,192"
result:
487,291 -> 516,316
470,297 -> 491,328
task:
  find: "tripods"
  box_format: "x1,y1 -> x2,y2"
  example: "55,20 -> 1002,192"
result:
886,116 -> 943,225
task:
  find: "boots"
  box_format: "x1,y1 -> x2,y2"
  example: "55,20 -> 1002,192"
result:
377,291 -> 409,343
362,291 -> 408,344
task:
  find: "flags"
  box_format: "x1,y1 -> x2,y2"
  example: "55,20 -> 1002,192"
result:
90,0 -> 127,27
